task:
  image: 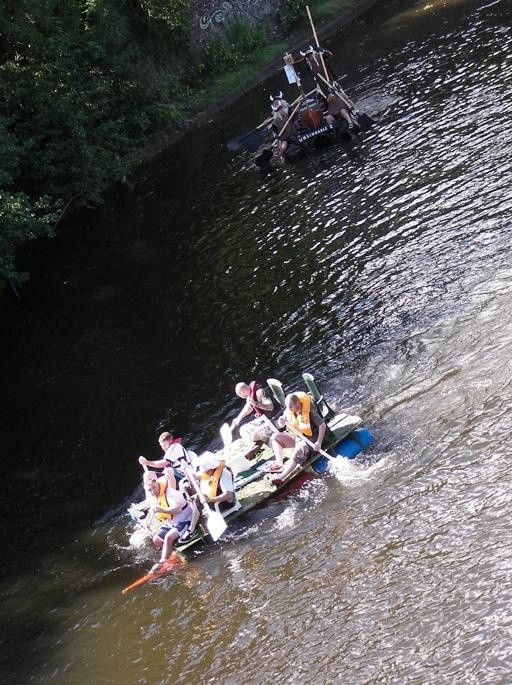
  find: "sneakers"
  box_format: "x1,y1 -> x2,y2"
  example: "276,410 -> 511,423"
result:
264,462 -> 283,472
149,561 -> 164,575
178,529 -> 198,544
245,446 -> 261,460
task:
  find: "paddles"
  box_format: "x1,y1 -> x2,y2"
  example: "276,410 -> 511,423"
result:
239,92 -> 302,145
220,405 -> 249,447
183,459 -> 227,541
256,100 -> 302,167
282,421 -> 352,470
317,72 -> 372,124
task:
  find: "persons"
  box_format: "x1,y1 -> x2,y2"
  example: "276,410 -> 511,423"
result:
263,391 -> 327,486
143,478 -> 195,576
271,97 -> 304,163
178,451 -> 234,544
138,432 -> 192,490
261,91 -> 296,124
326,83 -> 357,130
296,44 -> 342,110
232,381 -> 284,461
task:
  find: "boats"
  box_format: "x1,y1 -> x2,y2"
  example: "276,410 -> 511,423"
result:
128,373 -> 374,561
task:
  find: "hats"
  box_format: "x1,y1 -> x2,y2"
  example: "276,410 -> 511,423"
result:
200,455 -> 220,473
